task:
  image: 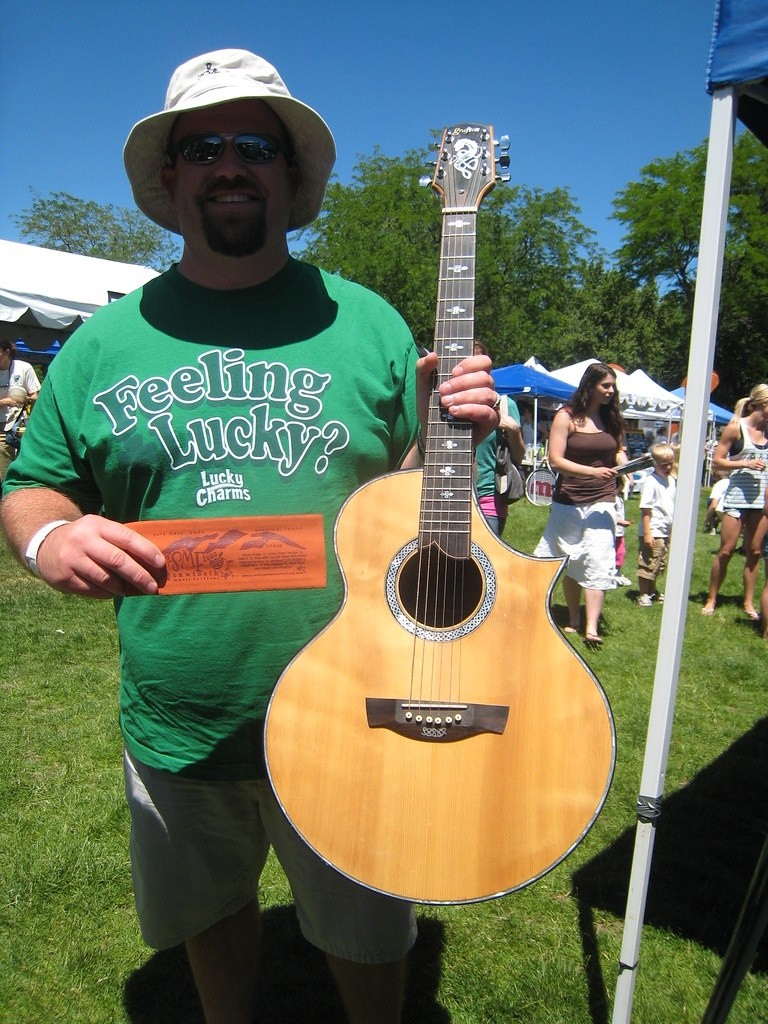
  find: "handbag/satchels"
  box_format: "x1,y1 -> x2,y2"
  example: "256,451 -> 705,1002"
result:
630,486 -> 634,494
495,395 -> 526,505
6,401 -> 30,448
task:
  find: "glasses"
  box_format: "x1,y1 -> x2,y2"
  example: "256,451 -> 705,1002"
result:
164,131 -> 290,164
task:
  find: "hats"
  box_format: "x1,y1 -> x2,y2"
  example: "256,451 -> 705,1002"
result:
123,49 -> 336,235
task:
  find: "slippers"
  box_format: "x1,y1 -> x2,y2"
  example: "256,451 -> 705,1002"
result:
702,608 -> 714,616
746,612 -> 759,620
586,633 -> 602,643
565,624 -> 581,632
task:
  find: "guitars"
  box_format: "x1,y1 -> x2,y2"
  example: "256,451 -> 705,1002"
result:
262,123 -> 619,911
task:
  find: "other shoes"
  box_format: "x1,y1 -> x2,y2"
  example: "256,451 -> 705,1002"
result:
710,528 -> 716,535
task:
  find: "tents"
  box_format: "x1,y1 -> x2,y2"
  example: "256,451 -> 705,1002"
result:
0,240 -> 162,370
488,356 -> 735,500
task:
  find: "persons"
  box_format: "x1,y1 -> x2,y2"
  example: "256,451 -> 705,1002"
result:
532,363 -> 635,646
613,383 -> 768,636
0,50 -> 500,1024
471,341 -> 526,541
0,336 -> 41,481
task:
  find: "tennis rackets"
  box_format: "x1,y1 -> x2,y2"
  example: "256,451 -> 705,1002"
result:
524,437 -> 556,507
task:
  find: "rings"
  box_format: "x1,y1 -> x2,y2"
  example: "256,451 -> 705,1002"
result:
492,391 -> 500,410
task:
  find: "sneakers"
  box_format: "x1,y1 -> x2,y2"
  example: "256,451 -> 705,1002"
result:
615,573 -> 631,586
652,591 -> 664,603
637,593 -> 656,606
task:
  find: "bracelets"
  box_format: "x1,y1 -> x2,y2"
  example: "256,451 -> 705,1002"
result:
417,431 -> 427,461
26,519 -> 72,578
